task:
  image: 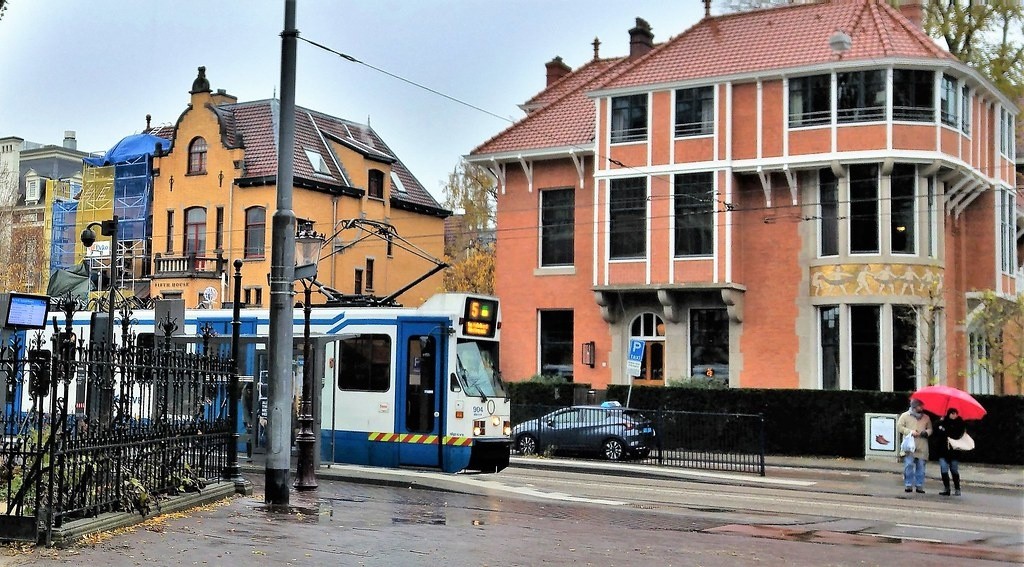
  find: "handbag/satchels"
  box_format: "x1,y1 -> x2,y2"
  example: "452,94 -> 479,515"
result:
947,430 -> 975,451
901,432 -> 916,453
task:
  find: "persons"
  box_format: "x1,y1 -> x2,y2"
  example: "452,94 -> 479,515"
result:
938,407 -> 965,496
898,398 -> 932,493
241,382 -> 262,462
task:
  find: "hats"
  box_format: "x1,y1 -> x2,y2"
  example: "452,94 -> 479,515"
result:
910,399 -> 922,408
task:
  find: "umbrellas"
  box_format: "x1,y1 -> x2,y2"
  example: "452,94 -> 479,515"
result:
909,386 -> 987,430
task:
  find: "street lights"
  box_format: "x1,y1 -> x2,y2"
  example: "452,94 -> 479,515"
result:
293,218 -> 324,492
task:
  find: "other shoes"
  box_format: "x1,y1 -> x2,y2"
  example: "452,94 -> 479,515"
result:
915,486 -> 925,493
905,487 -> 913,492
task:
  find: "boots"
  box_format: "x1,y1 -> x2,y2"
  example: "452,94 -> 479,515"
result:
938,472 -> 950,496
952,472 -> 961,496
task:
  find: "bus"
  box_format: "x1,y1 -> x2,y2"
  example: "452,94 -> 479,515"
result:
3,219 -> 512,475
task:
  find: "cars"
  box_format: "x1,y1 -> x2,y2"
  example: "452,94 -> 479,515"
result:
513,401 -> 655,462
